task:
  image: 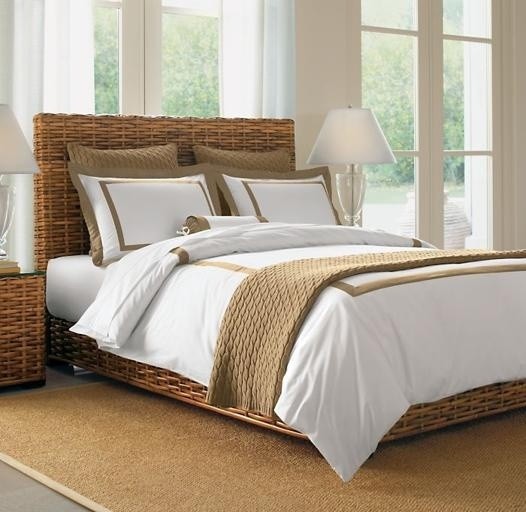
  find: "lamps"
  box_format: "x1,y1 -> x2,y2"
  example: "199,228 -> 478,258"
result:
307,104 -> 398,225
0,103 -> 41,260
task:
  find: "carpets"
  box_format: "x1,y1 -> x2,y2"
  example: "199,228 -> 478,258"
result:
1,377 -> 525,511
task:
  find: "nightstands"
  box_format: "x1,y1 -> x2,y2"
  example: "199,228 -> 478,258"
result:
0,273 -> 49,388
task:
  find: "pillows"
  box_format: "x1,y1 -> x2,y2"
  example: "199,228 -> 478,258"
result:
64,140 -> 343,269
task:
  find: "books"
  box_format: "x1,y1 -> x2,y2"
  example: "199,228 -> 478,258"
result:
1,266 -> 20,273
0,258 -> 18,269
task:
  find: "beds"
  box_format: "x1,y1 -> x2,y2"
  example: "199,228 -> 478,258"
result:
33,112 -> 526,459
